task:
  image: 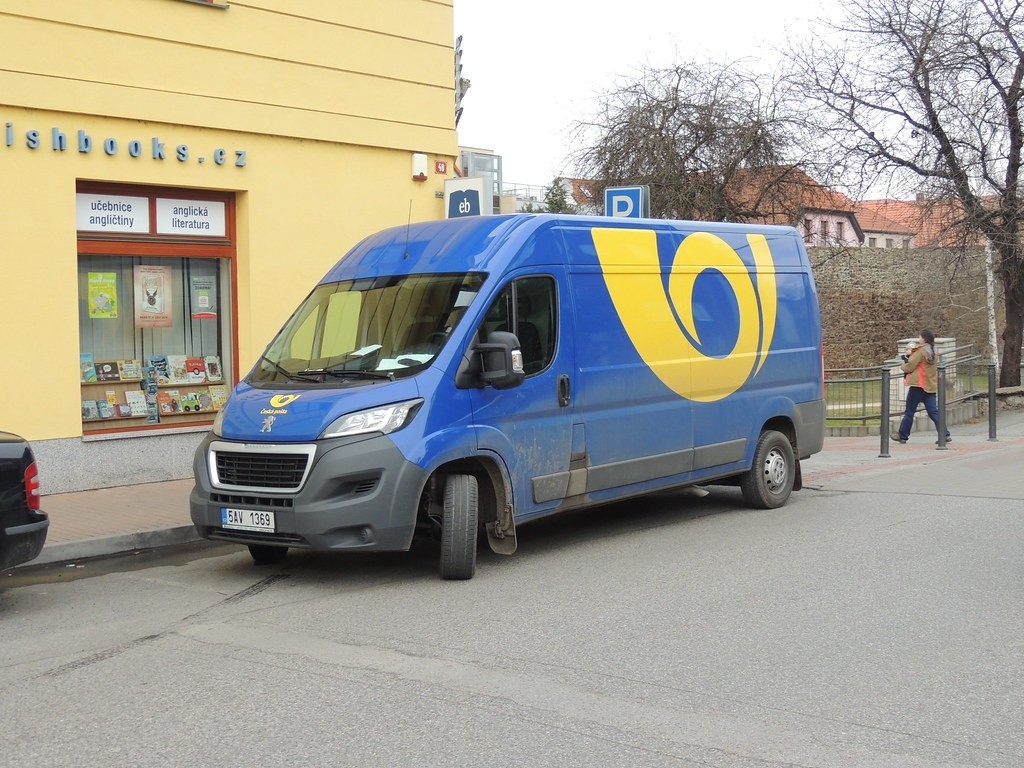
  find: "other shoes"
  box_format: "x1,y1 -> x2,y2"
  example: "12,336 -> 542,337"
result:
890,434 -> 906,443
936,436 -> 952,444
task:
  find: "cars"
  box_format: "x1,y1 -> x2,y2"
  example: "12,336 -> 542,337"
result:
0,427 -> 50,577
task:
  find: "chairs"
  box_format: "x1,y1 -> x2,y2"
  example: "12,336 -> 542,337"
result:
494,300 -> 542,374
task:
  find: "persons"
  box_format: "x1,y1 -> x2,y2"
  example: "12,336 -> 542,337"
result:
150,411 -> 155,420
901,341 -> 916,386
890,330 -> 952,444
171,399 -> 179,412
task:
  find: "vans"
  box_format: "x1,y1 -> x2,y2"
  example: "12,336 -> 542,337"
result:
189,214 -> 835,580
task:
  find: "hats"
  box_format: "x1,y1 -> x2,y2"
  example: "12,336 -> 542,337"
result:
905,342 -> 917,351
